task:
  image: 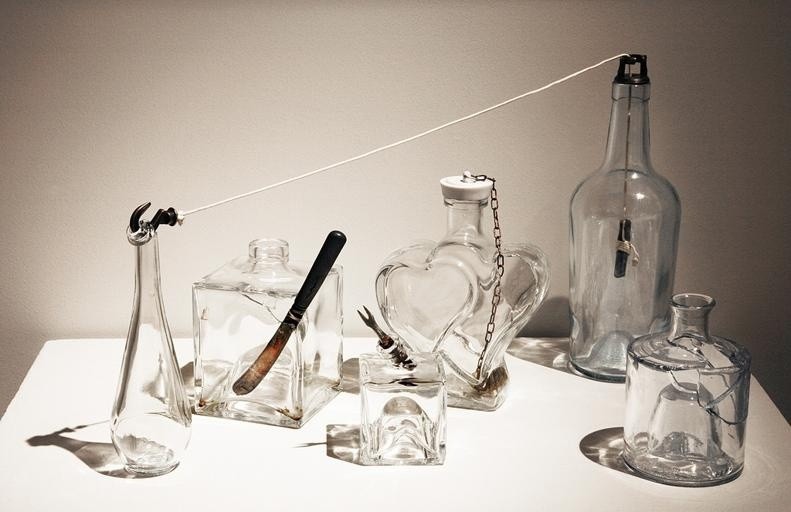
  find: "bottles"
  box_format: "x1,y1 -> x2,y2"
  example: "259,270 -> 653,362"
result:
570,54 -> 683,378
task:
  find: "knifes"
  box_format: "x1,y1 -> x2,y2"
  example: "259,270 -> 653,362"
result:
233,230 -> 347,395
